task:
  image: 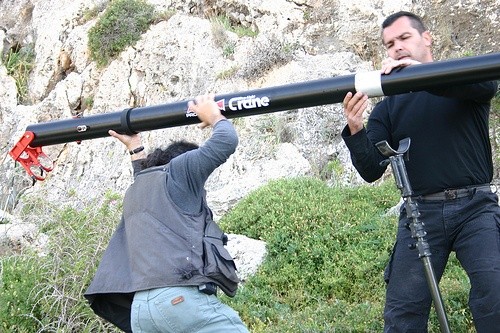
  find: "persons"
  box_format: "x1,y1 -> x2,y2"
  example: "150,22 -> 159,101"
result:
340,12 -> 500,333
83,94 -> 252,333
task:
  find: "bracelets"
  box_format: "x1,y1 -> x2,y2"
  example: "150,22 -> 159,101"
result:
129,146 -> 145,155
